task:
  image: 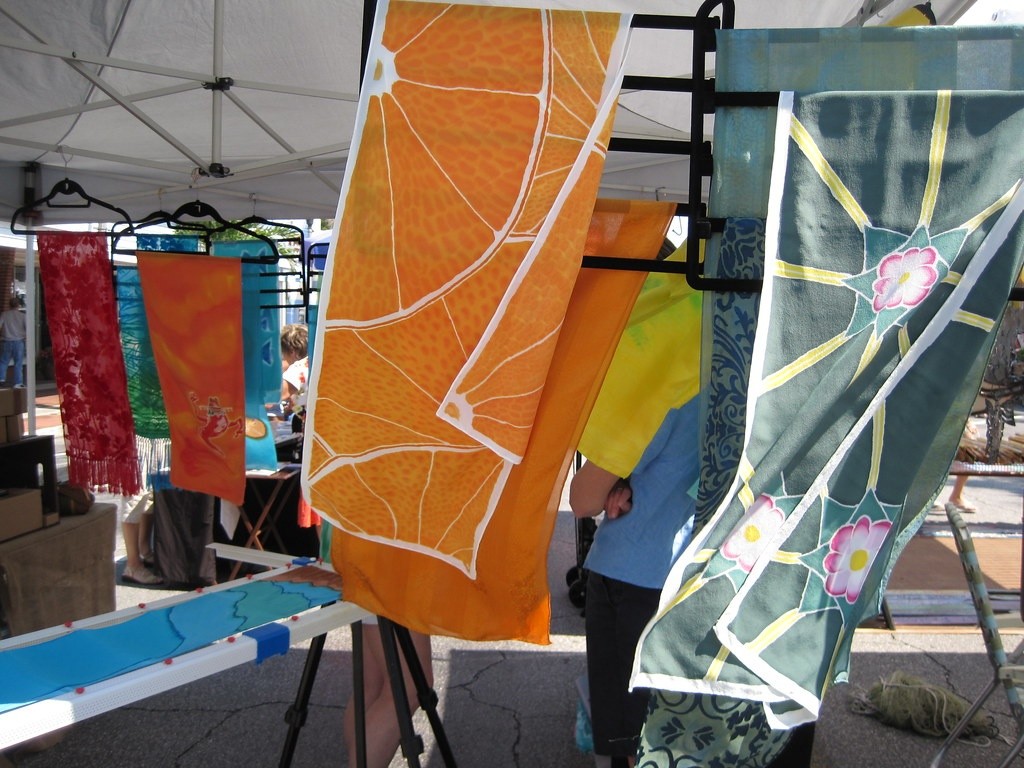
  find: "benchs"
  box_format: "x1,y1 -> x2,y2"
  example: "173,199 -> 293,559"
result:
0,559 -> 460,768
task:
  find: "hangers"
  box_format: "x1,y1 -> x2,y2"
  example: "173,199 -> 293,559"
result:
112,167 -> 279,264
110,187 -> 213,302
11,144 -> 133,236
207,193 -> 307,310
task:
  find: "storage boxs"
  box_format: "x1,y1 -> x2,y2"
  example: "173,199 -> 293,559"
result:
0,435 -> 61,526
0,502 -> 116,637
0,387 -> 28,443
0,488 -> 43,542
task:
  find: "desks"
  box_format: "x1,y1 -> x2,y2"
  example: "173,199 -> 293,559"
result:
226,469 -> 298,582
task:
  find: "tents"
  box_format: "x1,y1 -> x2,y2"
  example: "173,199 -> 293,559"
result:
0,0 -> 979,223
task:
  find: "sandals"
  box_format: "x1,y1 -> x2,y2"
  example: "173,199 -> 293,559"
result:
139,551 -> 155,566
122,562 -> 163,584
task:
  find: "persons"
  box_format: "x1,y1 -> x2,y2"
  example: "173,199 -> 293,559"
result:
343,614 -> 434,768
0,297 -> 27,389
276,320 -> 319,560
567,231 -> 702,768
926,415 -> 980,516
119,453 -> 166,587
